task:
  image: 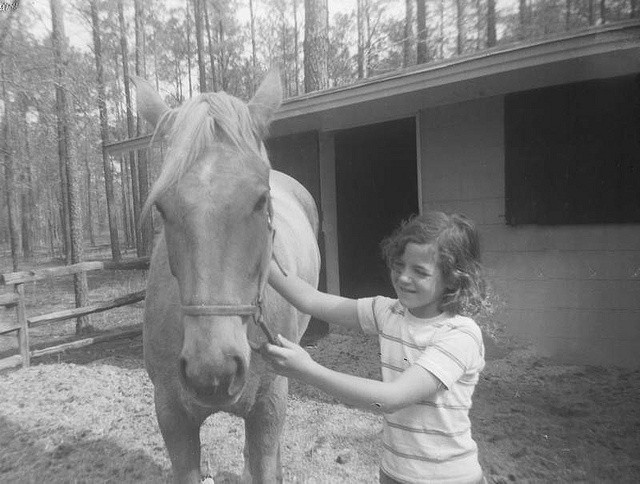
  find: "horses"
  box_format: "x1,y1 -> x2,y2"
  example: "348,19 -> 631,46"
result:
126,63 -> 324,484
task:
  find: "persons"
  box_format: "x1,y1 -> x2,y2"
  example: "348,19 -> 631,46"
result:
248,210 -> 485,484
